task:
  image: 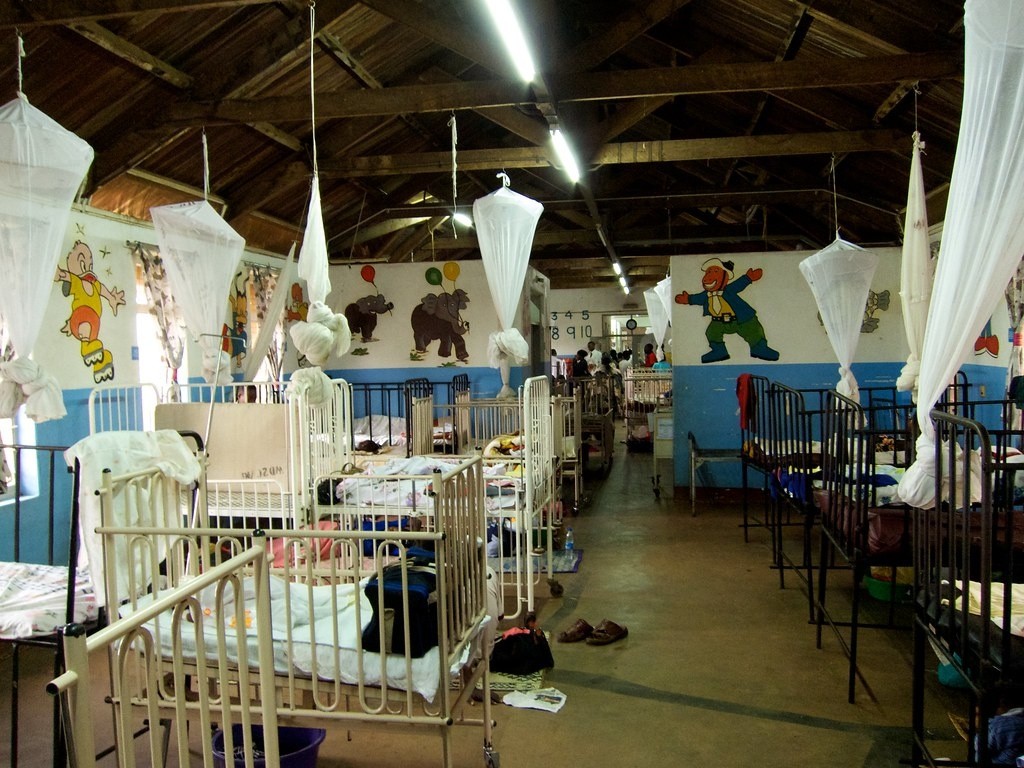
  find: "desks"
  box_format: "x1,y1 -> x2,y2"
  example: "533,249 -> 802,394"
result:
689,433 -> 743,517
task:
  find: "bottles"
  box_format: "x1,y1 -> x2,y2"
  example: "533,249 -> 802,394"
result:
565,528 -> 574,559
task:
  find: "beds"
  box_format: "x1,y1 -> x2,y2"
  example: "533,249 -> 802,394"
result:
736,373 -> 1023,768
0,367 -> 671,768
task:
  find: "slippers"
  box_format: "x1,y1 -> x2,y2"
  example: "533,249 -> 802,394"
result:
585,618 -> 628,647
557,618 -> 597,643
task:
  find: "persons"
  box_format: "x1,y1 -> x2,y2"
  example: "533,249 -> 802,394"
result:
236,383 -> 257,403
550,341 -> 658,417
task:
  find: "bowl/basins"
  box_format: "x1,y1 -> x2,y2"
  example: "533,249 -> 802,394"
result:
212,722 -> 326,768
862,576 -> 908,601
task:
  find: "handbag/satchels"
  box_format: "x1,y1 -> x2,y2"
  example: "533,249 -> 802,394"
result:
361,547 -> 466,658
490,625 -> 554,675
